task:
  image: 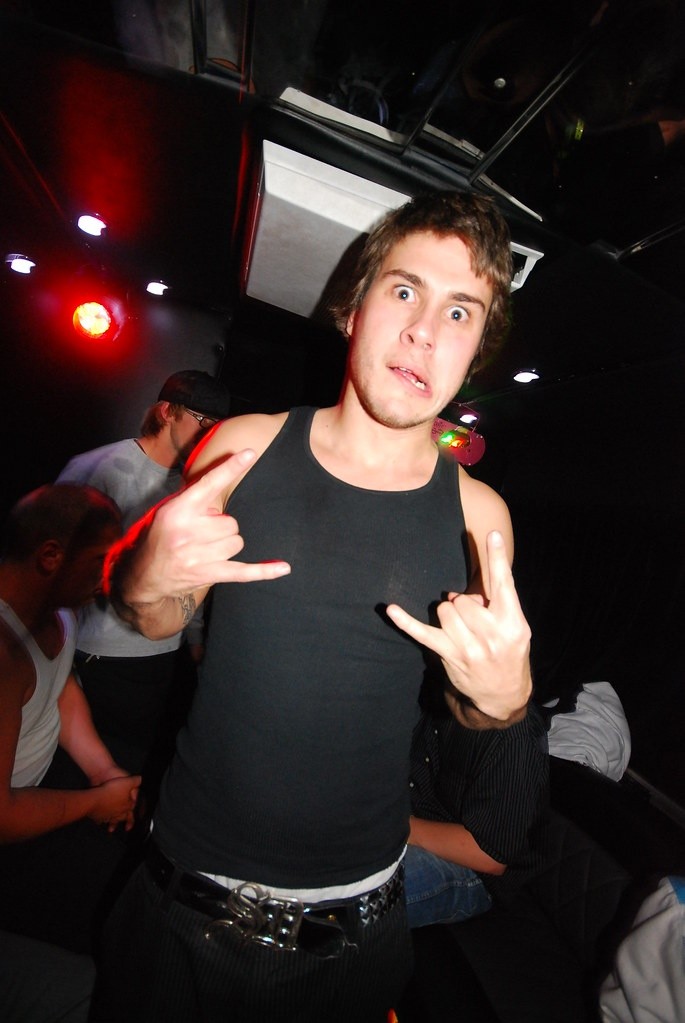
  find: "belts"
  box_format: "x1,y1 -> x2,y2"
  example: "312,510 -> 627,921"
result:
130,833 -> 406,961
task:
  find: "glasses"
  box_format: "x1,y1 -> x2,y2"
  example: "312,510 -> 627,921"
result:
184,409 -> 218,430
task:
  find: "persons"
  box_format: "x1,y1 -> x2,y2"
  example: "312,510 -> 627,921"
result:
402,694 -> 550,933
55,367 -> 232,713
122,185 -> 534,1023
0,481 -> 142,840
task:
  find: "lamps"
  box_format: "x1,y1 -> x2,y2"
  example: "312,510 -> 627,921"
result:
69,295 -> 123,345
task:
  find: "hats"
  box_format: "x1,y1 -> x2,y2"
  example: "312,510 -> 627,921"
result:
158,369 -> 231,424
